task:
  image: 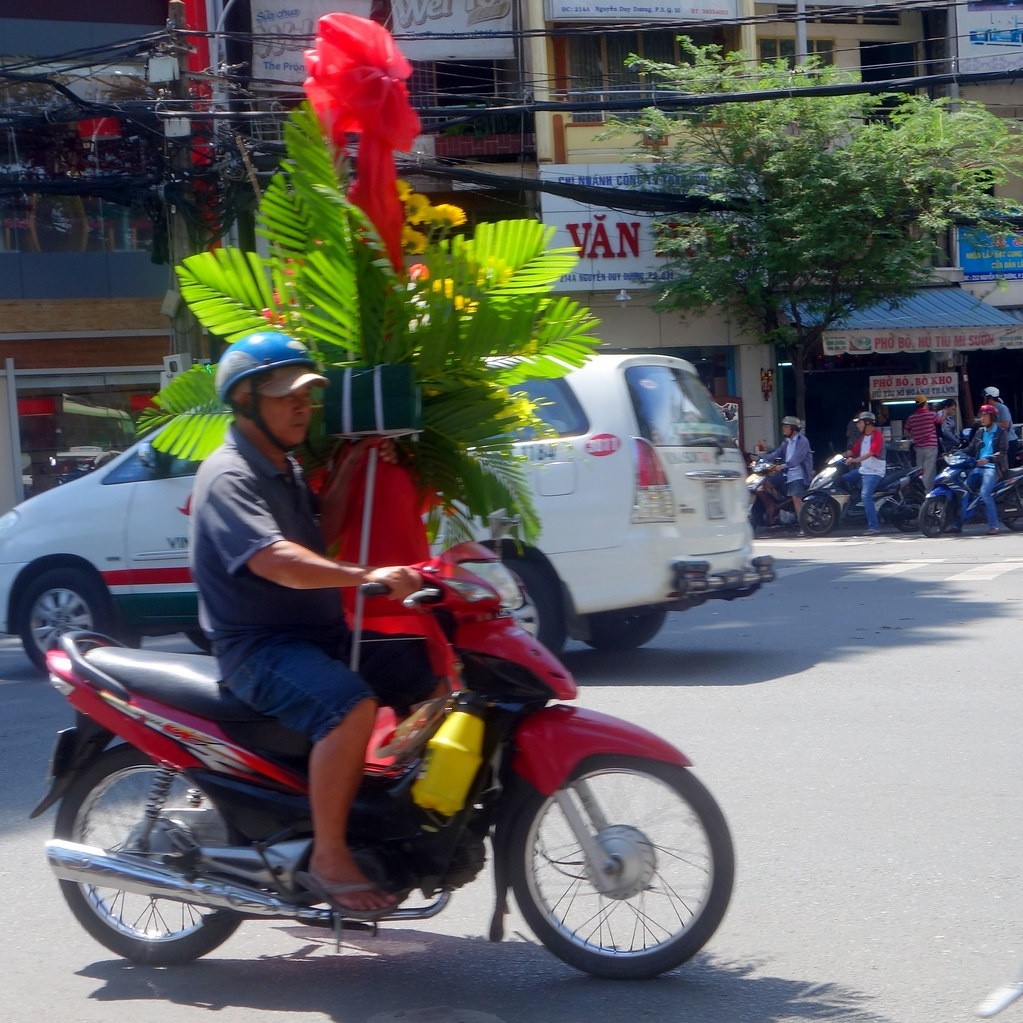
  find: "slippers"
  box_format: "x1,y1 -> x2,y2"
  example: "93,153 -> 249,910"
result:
294,871 -> 398,919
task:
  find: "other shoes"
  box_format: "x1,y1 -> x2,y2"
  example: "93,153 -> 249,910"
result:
862,528 -> 880,535
986,527 -> 1000,535
797,528 -> 807,537
936,525 -> 960,533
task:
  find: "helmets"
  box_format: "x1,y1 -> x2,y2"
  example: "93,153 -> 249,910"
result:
782,416 -> 801,429
979,405 -> 999,419
216,332 -> 314,404
983,387 -> 999,396
853,412 -> 875,422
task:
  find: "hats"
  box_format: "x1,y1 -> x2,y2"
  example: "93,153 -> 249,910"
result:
231,365 -> 329,397
915,395 -> 930,404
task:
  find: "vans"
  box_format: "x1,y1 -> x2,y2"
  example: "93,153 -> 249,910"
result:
0,356 -> 777,677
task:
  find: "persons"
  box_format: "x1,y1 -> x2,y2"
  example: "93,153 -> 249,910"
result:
903,395 -> 945,493
762,417 -> 809,536
185,325 -> 422,915
937,398 -> 961,448
843,412 -> 887,536
946,404 -> 1010,535
984,386 -> 1018,445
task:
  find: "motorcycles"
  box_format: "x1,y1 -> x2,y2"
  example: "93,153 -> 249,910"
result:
745,452 -> 815,531
799,452 -> 927,537
29,529 -> 739,980
915,435 -> 1023,537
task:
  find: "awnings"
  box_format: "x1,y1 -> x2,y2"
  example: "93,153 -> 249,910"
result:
778,286 -> 1023,353
